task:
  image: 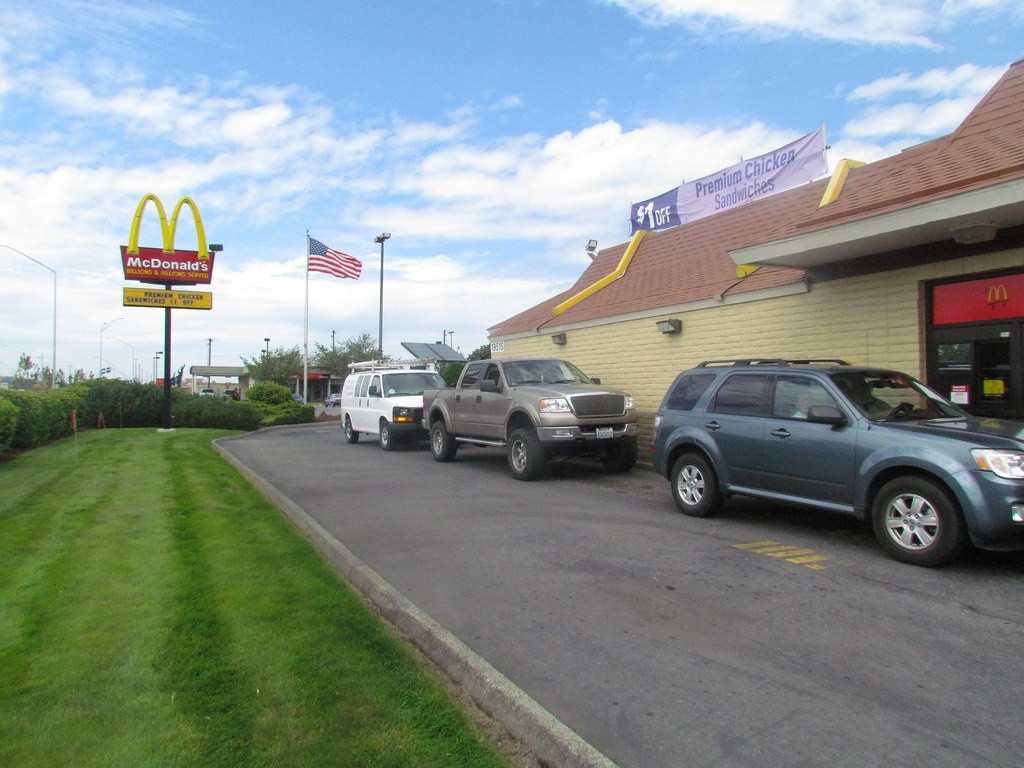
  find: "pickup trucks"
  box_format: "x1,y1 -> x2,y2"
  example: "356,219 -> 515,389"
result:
423,357 -> 641,482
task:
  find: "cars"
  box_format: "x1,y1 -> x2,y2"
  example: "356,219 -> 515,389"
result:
291,394 -> 304,404
324,392 -> 342,407
200,388 -> 216,399
221,389 -> 240,401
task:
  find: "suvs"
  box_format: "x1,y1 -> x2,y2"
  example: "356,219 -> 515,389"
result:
655,356 -> 1024,567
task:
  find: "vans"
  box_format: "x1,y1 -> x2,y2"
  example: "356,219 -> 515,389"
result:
341,360 -> 450,451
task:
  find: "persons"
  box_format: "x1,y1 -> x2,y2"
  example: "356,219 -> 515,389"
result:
852,382 -> 893,419
790,389 -> 813,418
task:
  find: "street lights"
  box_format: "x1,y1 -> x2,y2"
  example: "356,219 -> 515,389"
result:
154,357 -> 160,383
105,336 -> 135,379
264,337 -> 271,360
155,351 -> 164,383
375,232 -> 391,362
99,317 -> 125,379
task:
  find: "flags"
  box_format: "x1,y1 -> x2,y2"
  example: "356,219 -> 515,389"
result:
308,238 -> 362,280
